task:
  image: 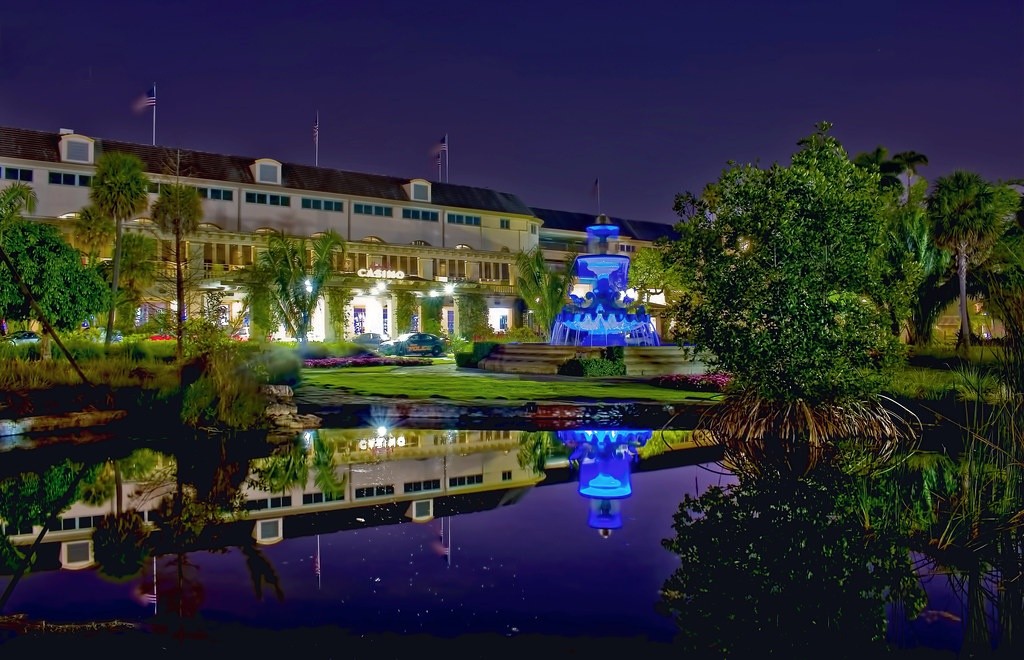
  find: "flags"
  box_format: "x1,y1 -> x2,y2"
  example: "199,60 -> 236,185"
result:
311,117 -> 317,142
129,90 -> 156,114
430,136 -> 447,165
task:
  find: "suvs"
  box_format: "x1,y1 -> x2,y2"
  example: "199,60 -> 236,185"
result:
624,332 -> 654,347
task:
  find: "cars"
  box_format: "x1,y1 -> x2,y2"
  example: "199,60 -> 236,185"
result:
147,333 -> 171,339
352,333 -> 390,349
82,327 -> 123,342
232,326 -> 275,342
1,331 -> 43,345
378,332 -> 447,357
